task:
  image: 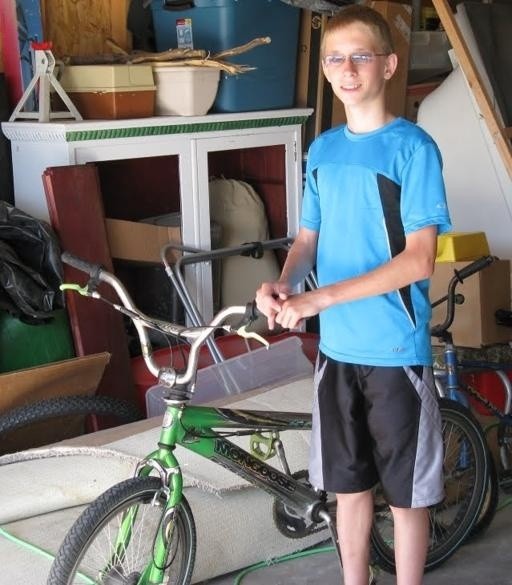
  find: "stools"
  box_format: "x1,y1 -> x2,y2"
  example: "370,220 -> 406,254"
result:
431,344 -> 512,470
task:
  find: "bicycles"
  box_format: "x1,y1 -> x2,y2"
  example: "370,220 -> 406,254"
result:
43,254 -> 488,585
415,253 -> 512,540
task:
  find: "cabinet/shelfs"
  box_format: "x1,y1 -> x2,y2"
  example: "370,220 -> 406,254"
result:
1,106 -> 314,354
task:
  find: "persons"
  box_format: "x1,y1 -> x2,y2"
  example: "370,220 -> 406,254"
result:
254,5 -> 453,585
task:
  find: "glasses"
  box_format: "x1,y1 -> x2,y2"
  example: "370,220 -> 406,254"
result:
324,53 -> 388,65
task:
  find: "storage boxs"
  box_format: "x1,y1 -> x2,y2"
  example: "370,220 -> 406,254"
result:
51,64 -> 154,121
145,333 -> 316,422
421,256 -> 511,348
146,0 -> 300,111
404,29 -> 456,124
105,216 -> 226,264
